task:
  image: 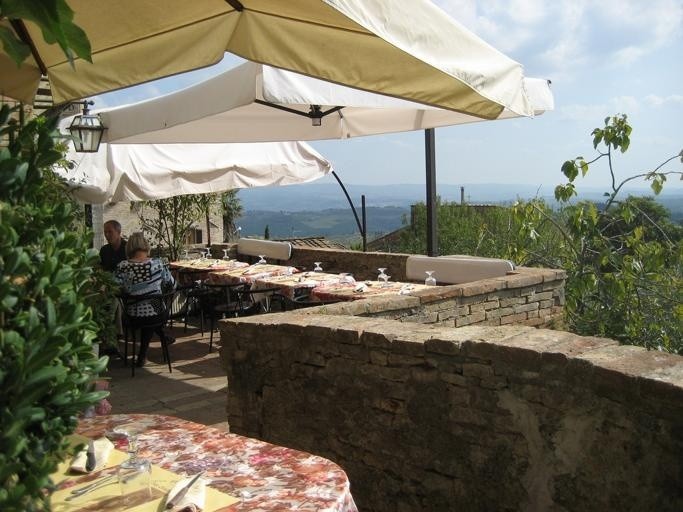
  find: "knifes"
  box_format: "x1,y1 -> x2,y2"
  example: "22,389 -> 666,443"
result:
165,470 -> 204,510
86,438 -> 95,473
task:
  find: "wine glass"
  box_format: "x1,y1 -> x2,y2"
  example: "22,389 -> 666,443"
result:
313,261 -> 323,276
113,422 -> 153,509
424,270 -> 437,290
198,246 -> 213,263
377,267 -> 388,286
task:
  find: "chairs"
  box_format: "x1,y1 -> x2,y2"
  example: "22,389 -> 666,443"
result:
114,263 -> 346,378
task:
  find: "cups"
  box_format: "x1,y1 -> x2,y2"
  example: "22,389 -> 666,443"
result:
222,249 -> 229,261
258,254 -> 266,266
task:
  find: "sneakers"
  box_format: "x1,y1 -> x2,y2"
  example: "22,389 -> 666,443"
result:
165,335 -> 175,344
136,356 -> 147,367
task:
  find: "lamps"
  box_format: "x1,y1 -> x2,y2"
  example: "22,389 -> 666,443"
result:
66,102 -> 108,152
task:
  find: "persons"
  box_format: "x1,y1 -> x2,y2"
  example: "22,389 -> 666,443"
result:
99,219 -> 129,355
112,231 -> 177,368
